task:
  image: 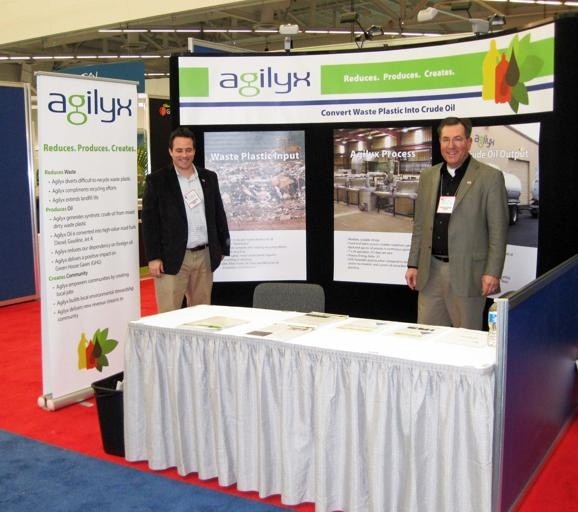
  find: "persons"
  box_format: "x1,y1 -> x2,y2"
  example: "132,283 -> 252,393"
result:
144,128 -> 230,314
404,118 -> 510,331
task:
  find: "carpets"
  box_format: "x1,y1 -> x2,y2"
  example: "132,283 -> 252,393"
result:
0,304 -> 315,512
513,416 -> 578,512
0,429 -> 299,512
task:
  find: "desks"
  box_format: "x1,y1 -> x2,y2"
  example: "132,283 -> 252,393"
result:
122,305 -> 497,512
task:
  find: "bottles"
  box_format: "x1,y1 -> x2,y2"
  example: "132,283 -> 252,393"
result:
488,298 -> 498,344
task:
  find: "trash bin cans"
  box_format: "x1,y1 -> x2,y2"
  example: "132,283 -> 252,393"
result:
91,371 -> 124,457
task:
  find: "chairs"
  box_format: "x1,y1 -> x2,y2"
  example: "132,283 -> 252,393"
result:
254,283 -> 325,314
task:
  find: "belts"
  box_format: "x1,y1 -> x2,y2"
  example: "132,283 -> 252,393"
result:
185,242 -> 209,252
433,255 -> 449,264
